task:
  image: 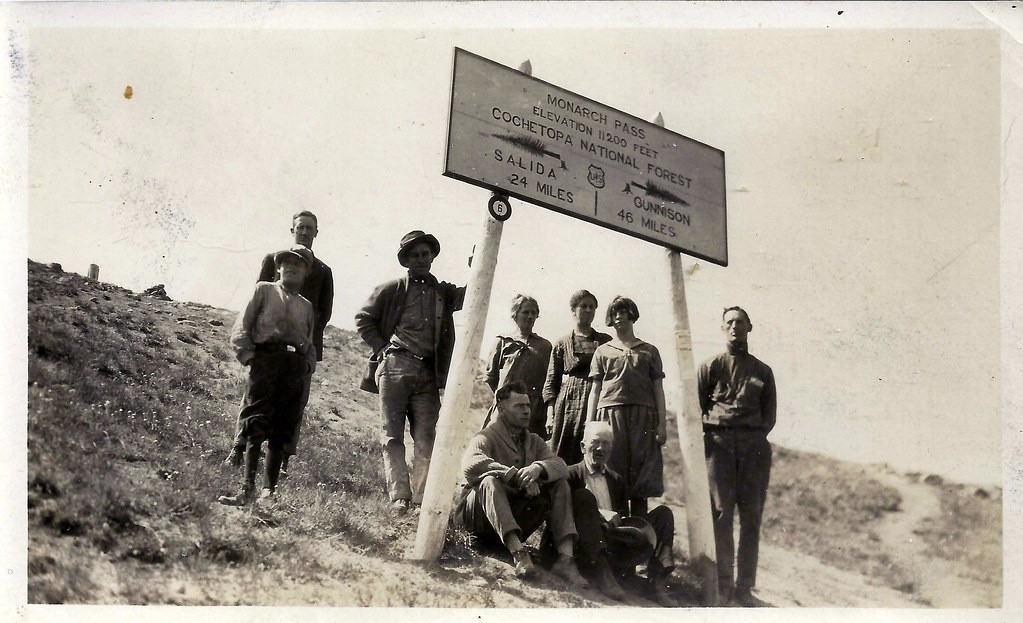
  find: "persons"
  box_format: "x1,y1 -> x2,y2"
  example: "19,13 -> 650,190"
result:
225,209 -> 334,482
355,230 -> 476,513
696,306 -> 777,607
455,289 -> 681,607
230,243 -> 317,508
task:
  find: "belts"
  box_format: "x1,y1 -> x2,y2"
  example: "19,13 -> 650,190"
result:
286,345 -> 297,353
399,349 -> 424,360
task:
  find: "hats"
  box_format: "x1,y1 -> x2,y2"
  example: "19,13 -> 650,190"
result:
397,230 -> 441,268
605,516 -> 657,562
274,244 -> 315,268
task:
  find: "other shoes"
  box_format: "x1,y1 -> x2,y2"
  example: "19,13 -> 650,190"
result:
735,585 -> 756,607
597,566 -> 626,599
412,502 -> 421,511
512,544 -> 536,578
551,553 -> 589,588
391,498 -> 409,512
218,481 -> 258,505
221,446 -> 246,469
647,579 -> 671,607
719,584 -> 733,606
256,487 -> 275,504
278,459 -> 288,478
